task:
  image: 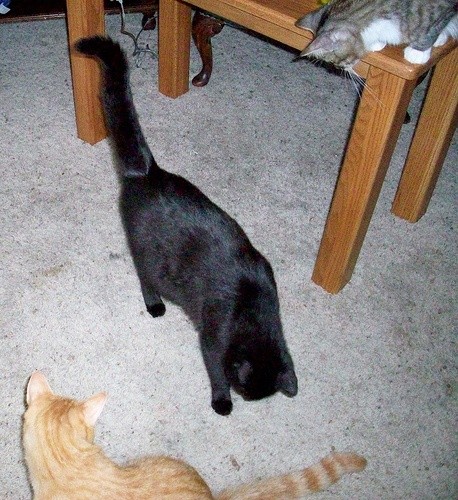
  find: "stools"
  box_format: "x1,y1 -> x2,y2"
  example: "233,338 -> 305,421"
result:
64,0 -> 457,297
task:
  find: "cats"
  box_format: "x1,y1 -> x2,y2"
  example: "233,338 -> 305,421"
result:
293,0 -> 458,114
19,371 -> 368,500
73,32 -> 298,417
